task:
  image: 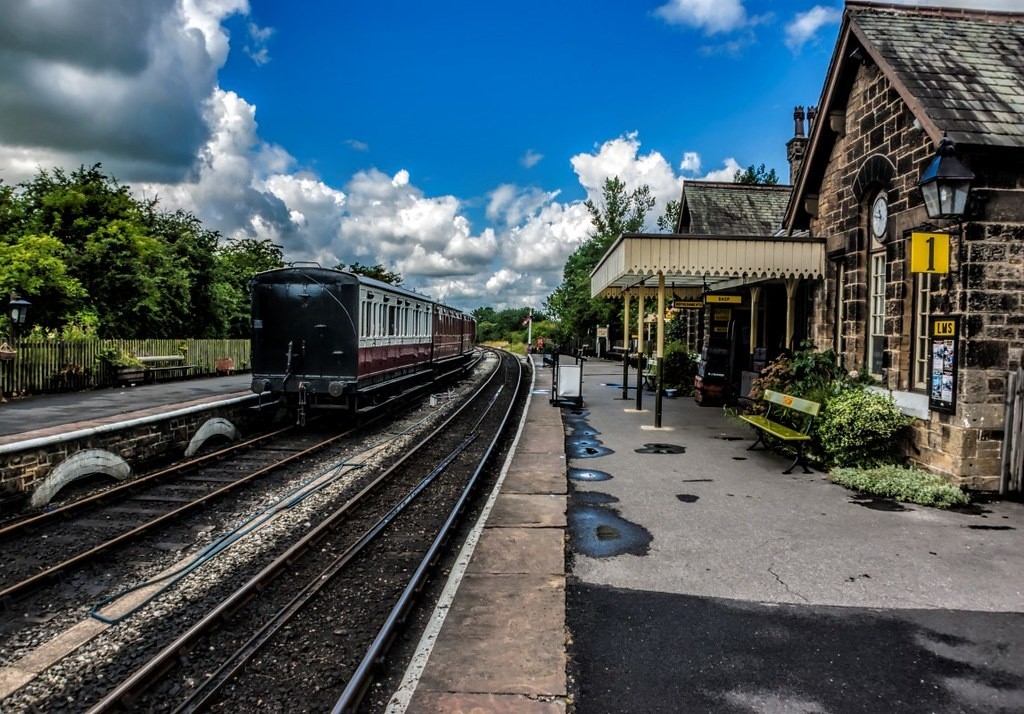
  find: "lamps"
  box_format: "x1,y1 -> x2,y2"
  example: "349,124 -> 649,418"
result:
915,126 -> 976,219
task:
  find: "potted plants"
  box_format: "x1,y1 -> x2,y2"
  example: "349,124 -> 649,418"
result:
111,356 -> 144,386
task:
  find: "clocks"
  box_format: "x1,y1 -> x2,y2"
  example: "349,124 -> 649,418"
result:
870,190 -> 887,243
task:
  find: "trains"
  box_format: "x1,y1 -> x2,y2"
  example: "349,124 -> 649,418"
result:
251,261 -> 477,426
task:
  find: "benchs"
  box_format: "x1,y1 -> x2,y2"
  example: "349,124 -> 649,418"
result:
136,354 -> 196,384
642,360 -> 657,391
739,389 -> 821,474
